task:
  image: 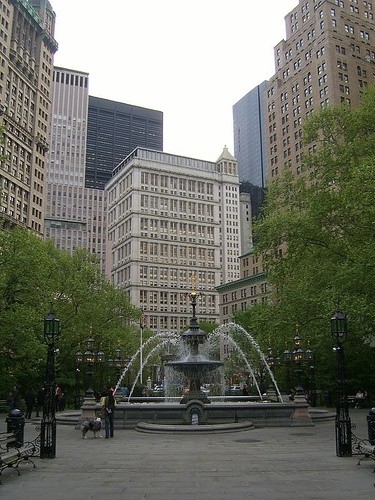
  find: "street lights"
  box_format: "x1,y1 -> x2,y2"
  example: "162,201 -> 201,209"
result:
75,334 -> 106,398
259,345 -> 280,384
281,334 -> 314,398
39,309 -> 63,458
107,347 -> 130,390
329,309 -> 352,456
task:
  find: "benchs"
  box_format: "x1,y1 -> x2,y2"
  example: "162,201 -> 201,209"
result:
357,439 -> 375,472
0,430 -> 37,485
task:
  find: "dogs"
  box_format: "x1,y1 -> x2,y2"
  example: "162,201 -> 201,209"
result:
81,416 -> 103,439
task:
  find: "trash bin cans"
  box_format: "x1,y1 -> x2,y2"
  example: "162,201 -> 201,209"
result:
367,408 -> 375,442
5,409 -> 25,448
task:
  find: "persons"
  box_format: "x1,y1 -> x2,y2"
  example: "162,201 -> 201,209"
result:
101,387 -> 116,439
6,385 -> 65,419
354,390 -> 369,410
